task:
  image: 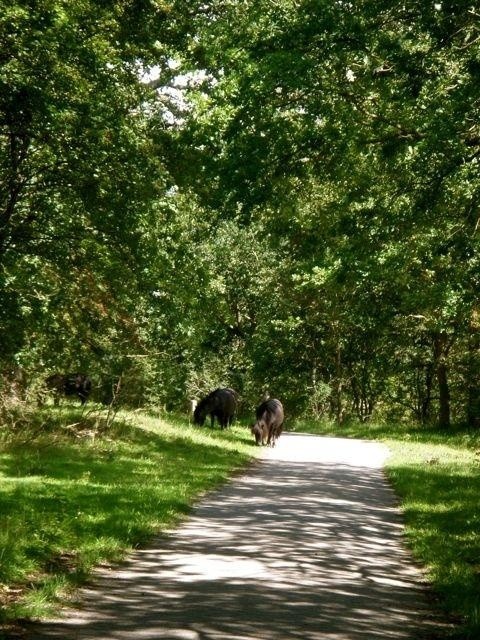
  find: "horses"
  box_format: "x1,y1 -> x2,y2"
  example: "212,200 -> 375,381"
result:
194,388 -> 237,430
251,399 -> 284,445
36,372 -> 91,406
